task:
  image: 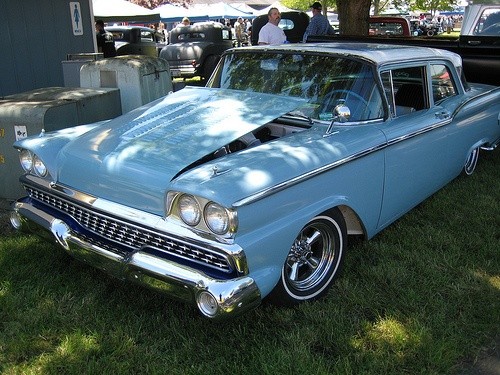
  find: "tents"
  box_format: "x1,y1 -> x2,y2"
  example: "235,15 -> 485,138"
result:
379,8 -> 410,16
92,0 -> 338,33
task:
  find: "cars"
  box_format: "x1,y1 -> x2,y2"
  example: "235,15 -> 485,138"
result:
105,27 -> 167,57
369,14 -> 411,36
10,43 -> 500,322
459,0 -> 500,43
250,11 -> 310,46
158,21 -> 233,81
411,19 -> 436,37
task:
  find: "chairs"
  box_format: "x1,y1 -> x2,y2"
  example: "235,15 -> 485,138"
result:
395,84 -> 425,110
327,80 -> 357,100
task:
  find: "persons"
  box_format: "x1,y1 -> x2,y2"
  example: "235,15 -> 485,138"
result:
301,2 -> 336,43
418,13 -> 463,33
146,17 -> 252,47
257,7 -> 288,93
95,19 -> 118,56
